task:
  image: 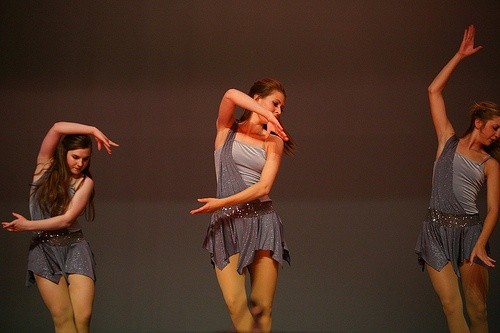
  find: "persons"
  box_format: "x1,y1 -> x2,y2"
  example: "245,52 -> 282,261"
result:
190,77 -> 292,333
1,121 -> 121,333
414,24 -> 500,333
